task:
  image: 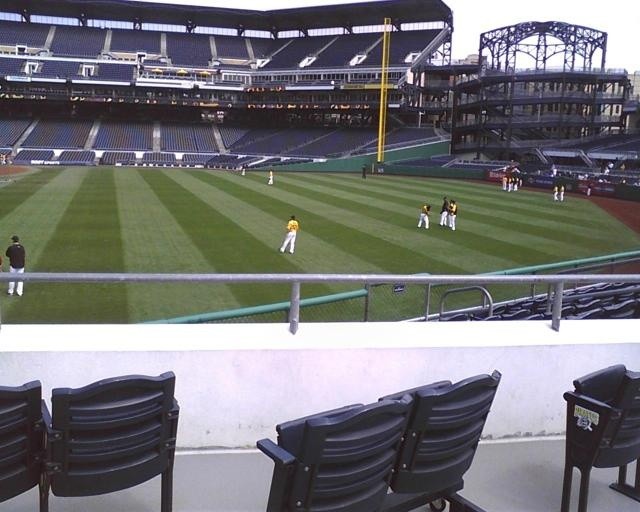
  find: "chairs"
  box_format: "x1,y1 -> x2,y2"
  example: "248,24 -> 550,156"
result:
392,153 -> 638,199
427,278 -> 640,325
1,21 -> 442,91
40,369 -> 182,512
0,100 -> 439,167
0,371 -> 46,512
553,361 -> 640,512
256,363 -> 503,512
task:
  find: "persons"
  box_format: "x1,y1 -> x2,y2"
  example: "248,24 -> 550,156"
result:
267,170 -> 274,186
587,183 -> 591,196
553,186 -> 559,201
524,160 -> 640,186
417,203 -> 431,229
559,185 -> 565,203
500,159 -> 523,192
5,235 -> 25,297
279,215 -> 299,254
240,163 -> 246,176
440,197 -> 449,228
448,200 -> 458,230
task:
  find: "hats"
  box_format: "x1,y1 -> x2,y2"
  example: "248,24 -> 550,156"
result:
11,236 -> 19,241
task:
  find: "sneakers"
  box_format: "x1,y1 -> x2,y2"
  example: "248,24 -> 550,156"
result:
7,293 -> 23,297
